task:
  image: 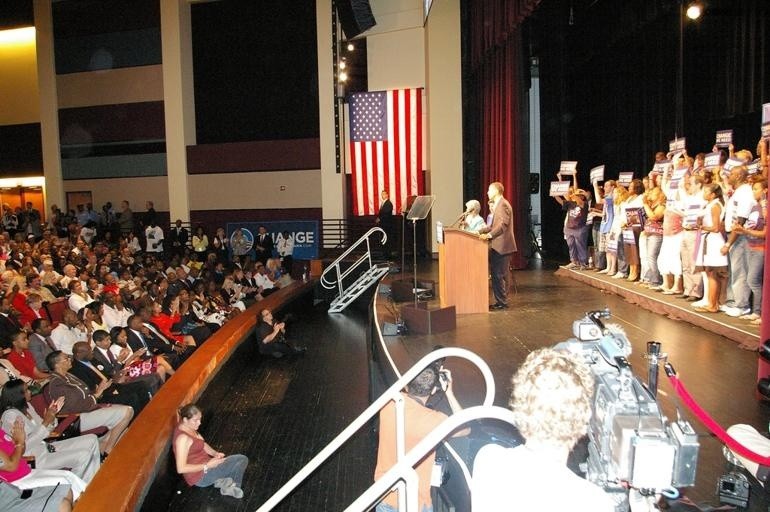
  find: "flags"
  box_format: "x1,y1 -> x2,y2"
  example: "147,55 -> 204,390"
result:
346,88 -> 424,217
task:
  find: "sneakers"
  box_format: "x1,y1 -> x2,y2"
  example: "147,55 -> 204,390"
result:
220,488 -> 244,499
214,478 -> 232,488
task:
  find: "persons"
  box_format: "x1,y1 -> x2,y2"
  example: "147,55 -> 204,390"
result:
1,331 -> 215,511
173,403 -> 249,499
459,199 -> 487,236
1,199 -> 294,331
373,365 -> 471,512
477,182 -> 518,309
487,201 -> 495,227
256,308 -> 307,358
722,423 -> 770,490
471,346 -> 614,512
375,190 -> 393,261
555,137 -> 769,325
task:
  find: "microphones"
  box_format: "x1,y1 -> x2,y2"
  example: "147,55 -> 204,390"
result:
466,208 -> 474,214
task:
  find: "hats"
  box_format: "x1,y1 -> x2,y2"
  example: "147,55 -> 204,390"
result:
574,189 -> 588,197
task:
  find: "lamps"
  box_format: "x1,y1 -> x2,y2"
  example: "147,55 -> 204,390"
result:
685,0 -> 708,21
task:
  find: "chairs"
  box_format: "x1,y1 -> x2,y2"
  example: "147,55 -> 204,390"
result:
31,381 -> 109,437
42,297 -> 69,327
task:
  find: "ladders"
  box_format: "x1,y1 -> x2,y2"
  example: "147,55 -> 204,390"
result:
320,227 -> 390,313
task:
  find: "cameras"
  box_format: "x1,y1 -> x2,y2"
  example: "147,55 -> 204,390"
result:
719,471 -> 750,508
429,345 -> 446,388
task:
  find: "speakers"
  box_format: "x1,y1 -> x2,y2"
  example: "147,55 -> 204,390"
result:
334,0 -> 377,40
382,315 -> 397,336
339,35 -> 368,103
380,282 -> 392,294
529,172 -> 539,194
390,266 -> 400,272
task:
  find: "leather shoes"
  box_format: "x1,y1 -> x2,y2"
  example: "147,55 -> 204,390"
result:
565,262 -> 762,326
491,303 -> 509,311
